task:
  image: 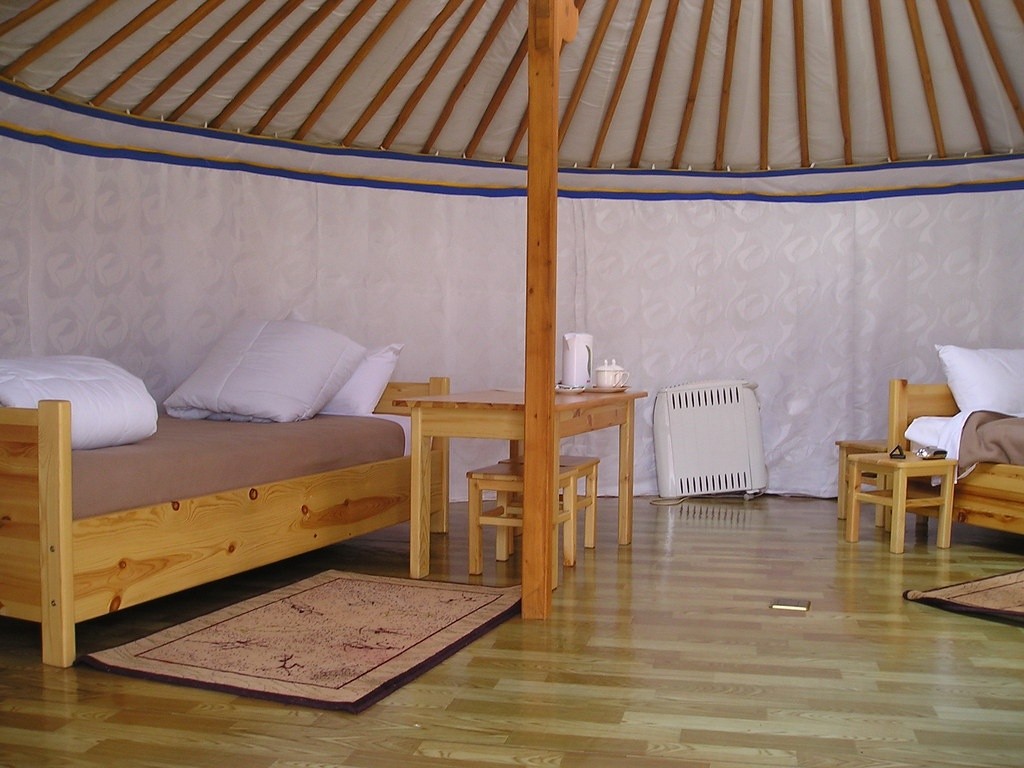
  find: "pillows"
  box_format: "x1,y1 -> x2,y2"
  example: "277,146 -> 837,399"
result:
936,344 -> 1024,416
319,336 -> 404,418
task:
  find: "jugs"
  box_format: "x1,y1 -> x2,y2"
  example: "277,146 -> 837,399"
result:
561,333 -> 594,388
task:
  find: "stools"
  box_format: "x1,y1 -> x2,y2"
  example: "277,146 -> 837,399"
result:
835,438 -> 889,527
499,453 -> 601,566
845,453 -> 958,554
466,464 -> 578,576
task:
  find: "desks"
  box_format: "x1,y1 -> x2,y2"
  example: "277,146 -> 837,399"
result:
393,388 -> 650,581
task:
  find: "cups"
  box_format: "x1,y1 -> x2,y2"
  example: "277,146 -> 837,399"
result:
609,358 -> 629,388
595,360 -> 623,388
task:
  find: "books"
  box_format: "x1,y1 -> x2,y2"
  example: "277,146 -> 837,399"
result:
769,598 -> 811,611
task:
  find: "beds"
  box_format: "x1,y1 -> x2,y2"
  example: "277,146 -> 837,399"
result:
885,378 -> 1024,536
0,375 -> 452,668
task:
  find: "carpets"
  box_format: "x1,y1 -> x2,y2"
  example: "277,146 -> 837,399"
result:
903,568 -> 1024,628
73,567 -> 524,718
